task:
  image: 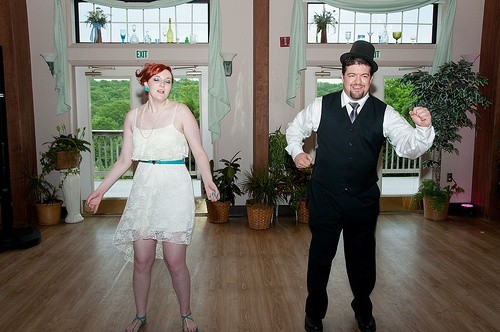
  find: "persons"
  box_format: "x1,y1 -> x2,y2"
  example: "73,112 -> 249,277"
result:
284,40 -> 436,331
86,65 -> 221,332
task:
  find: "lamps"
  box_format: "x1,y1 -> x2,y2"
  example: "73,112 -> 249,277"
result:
219,50 -> 237,76
41,52 -> 58,77
461,53 -> 480,68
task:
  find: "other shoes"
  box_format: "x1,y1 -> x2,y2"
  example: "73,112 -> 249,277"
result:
304,319 -> 323,332
355,313 -> 376,332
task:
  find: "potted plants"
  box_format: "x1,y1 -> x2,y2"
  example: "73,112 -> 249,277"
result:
15,171 -> 67,226
199,151 -> 244,223
268,125 -> 313,223
39,122 -> 91,170
395,59 -> 492,221
237,164 -> 283,230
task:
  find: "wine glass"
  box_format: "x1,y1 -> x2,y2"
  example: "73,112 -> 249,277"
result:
393,32 -> 401,44
377,32 -> 382,43
345,32 -> 351,44
367,32 -> 374,42
410,33 -> 415,44
120,29 -> 126,44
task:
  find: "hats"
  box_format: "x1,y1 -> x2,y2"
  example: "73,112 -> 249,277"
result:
339,40 -> 379,76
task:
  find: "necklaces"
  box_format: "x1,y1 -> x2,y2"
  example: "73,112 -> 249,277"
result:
138,99 -> 168,139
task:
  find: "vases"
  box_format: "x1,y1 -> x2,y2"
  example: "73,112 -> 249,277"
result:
321,27 -> 327,43
97,29 -> 102,43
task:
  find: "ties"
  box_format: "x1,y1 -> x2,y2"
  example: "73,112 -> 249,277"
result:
349,102 -> 360,124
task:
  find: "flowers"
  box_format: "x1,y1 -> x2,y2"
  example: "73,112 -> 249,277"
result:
84,9 -> 111,31
309,8 -> 338,43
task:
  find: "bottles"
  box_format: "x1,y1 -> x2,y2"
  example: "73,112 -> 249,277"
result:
382,27 -> 389,43
166,18 -> 173,43
130,25 -> 139,44
357,35 -> 365,41
185,37 -> 189,42
144,31 -> 151,43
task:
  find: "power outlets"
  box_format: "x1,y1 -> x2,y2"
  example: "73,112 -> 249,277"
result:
447,173 -> 452,182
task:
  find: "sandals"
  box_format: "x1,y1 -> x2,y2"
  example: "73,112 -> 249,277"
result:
181,313 -> 198,332
125,314 -> 146,332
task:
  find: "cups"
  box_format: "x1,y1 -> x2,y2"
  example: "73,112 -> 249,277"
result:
191,35 -> 198,43
155,39 -> 160,43
